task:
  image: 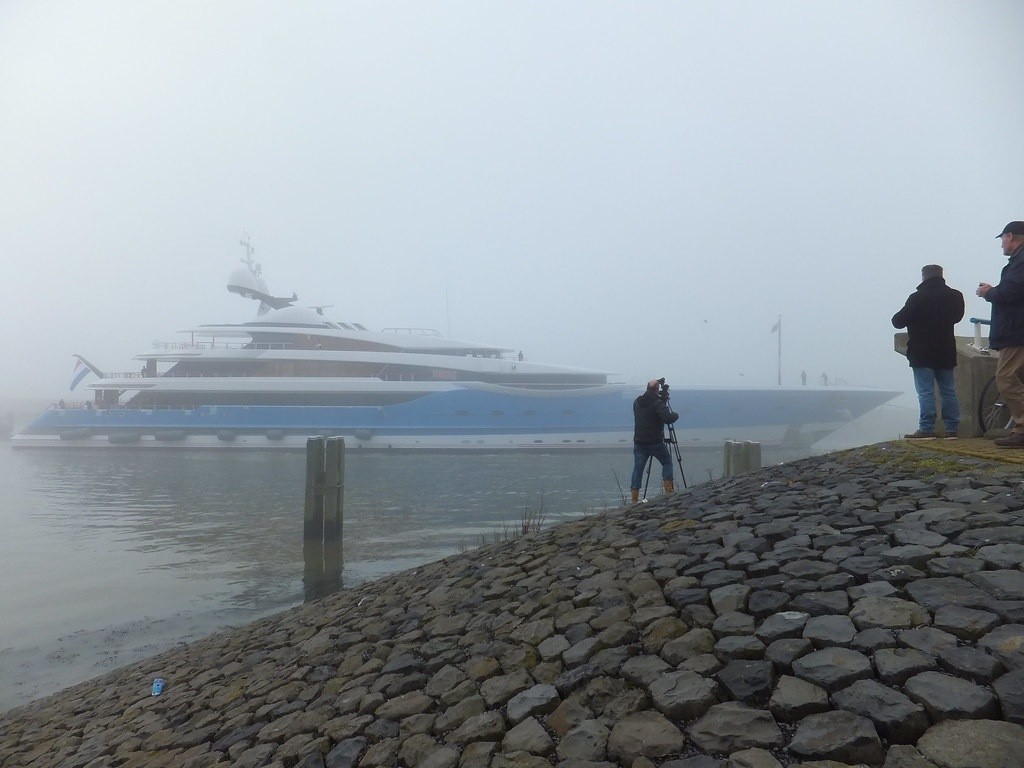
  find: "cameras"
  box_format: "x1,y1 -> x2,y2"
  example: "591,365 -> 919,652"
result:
657,378 -> 669,390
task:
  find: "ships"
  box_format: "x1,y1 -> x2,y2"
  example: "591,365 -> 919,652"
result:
10,234 -> 905,453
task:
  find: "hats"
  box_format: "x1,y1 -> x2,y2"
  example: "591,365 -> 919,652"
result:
995,221 -> 1024,238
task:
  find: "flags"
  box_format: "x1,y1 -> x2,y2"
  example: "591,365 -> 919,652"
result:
771,323 -> 778,333
69,360 -> 90,391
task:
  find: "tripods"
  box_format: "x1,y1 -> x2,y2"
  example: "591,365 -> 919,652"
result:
643,390 -> 687,500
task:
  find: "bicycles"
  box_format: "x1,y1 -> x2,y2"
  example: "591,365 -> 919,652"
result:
977,375 -> 1015,433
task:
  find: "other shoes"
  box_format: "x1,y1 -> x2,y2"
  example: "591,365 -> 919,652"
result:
944,432 -> 958,440
993,431 -> 1024,449
904,430 -> 936,440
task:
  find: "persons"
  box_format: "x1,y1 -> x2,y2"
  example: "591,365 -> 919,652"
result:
976,221 -> 1024,449
59,399 -> 64,409
822,373 -> 827,385
85,400 -> 92,409
518,351 -> 523,361
141,365 -> 146,377
801,371 -> 806,385
630,380 -> 679,504
891,265 -> 965,439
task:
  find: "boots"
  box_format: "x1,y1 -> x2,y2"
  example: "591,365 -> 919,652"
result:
630,487 -> 639,503
663,479 -> 674,494
